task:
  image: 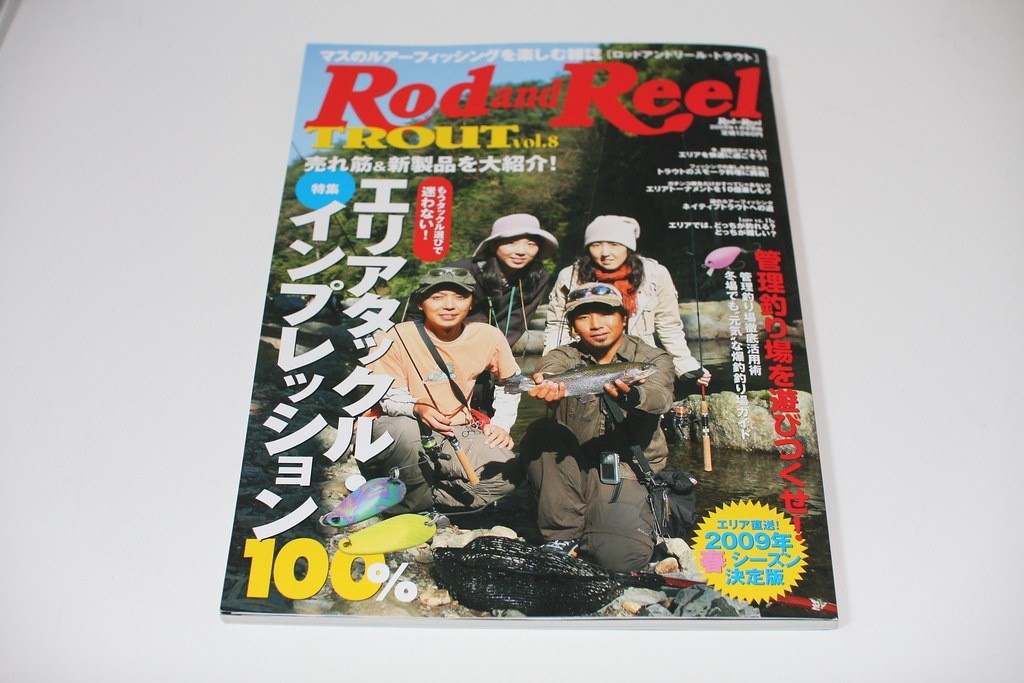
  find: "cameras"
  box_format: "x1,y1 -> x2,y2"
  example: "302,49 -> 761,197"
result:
599,452 -> 620,484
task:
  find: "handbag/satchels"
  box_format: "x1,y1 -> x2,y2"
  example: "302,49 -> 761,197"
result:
650,463 -> 699,542
470,408 -> 491,430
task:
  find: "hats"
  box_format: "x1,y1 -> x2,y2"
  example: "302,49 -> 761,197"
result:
584,215 -> 641,252
409,266 -> 476,304
472,213 -> 559,260
564,282 -> 623,318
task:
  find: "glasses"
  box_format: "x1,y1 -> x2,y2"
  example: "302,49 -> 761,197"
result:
565,286 -> 616,299
425,269 -> 470,277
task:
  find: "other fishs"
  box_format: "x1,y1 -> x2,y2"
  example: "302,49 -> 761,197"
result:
505,361 -> 659,404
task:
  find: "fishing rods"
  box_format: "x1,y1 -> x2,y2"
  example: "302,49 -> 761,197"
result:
682,95 -> 718,471
290,139 -> 479,490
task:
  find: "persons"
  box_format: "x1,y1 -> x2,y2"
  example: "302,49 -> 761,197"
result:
352,264 -> 521,529
541,215 -> 712,388
519,283 -> 676,575
439,214 -> 559,348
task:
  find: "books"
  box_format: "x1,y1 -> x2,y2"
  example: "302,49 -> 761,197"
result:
220,44 -> 837,618
